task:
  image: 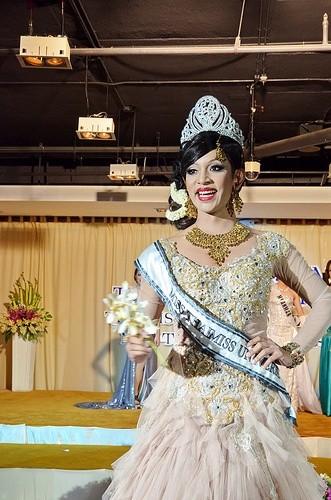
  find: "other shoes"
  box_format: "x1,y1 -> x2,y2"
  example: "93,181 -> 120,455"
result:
135,393 -> 140,408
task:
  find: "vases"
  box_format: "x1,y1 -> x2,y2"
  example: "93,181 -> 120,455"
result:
12,332 -> 36,392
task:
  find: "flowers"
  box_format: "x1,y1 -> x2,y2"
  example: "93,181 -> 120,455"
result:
103,281 -> 169,367
0,272 -> 52,353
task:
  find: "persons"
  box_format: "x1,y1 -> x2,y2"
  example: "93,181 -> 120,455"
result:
266,277 -> 323,416
318,260 -> 331,418
73,266 -> 166,411
99,94 -> 331,500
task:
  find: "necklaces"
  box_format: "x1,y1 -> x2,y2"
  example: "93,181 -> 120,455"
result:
183,221 -> 255,266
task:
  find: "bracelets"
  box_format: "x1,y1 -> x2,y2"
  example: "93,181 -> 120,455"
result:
279,343 -> 305,369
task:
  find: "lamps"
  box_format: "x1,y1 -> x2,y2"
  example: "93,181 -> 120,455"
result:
299,123 -> 323,152
107,108 -> 140,181
75,56 -> 116,141
16,0 -> 72,69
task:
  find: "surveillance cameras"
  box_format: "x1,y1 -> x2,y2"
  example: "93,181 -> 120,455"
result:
245,162 -> 260,181
